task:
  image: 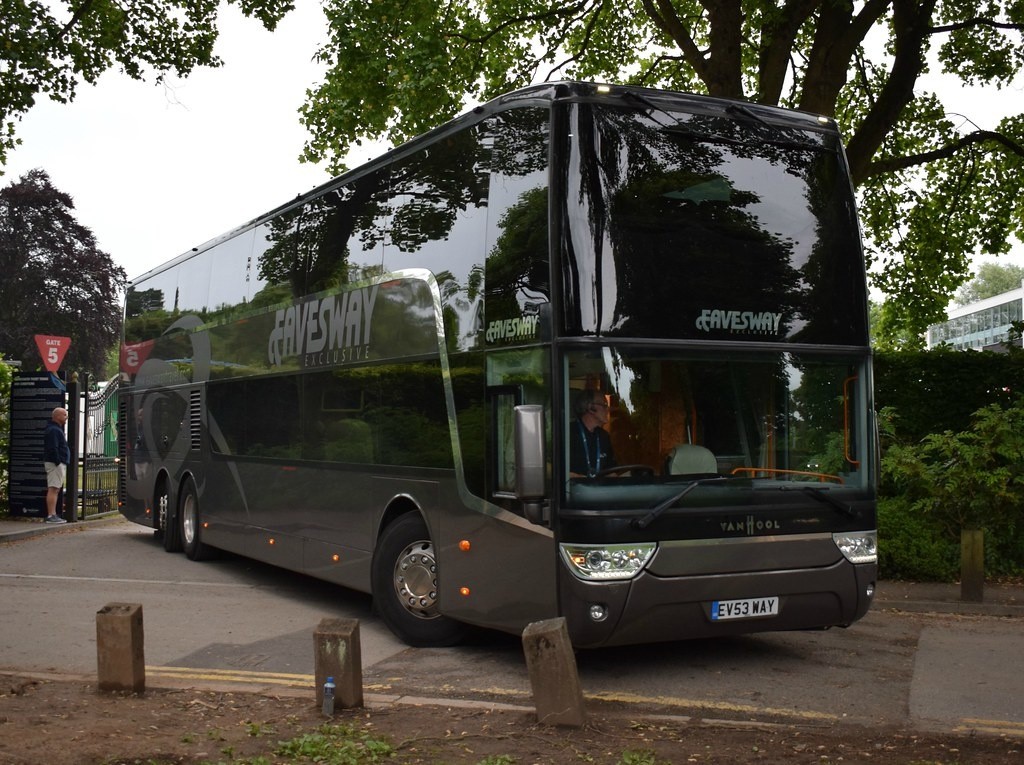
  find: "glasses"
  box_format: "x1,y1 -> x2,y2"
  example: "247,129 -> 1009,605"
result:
591,402 -> 609,409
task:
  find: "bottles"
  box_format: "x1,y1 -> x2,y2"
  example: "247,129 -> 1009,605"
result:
322,676 -> 335,717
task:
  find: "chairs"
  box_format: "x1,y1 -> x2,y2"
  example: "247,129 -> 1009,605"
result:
666,444 -> 717,476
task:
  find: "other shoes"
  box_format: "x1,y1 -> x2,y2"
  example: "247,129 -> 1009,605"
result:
46,515 -> 67,523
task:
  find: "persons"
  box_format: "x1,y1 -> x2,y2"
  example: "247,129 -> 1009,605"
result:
570,389 -> 618,478
43,407 -> 71,524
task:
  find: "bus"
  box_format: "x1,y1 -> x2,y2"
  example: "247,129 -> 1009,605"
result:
114,80 -> 882,651
